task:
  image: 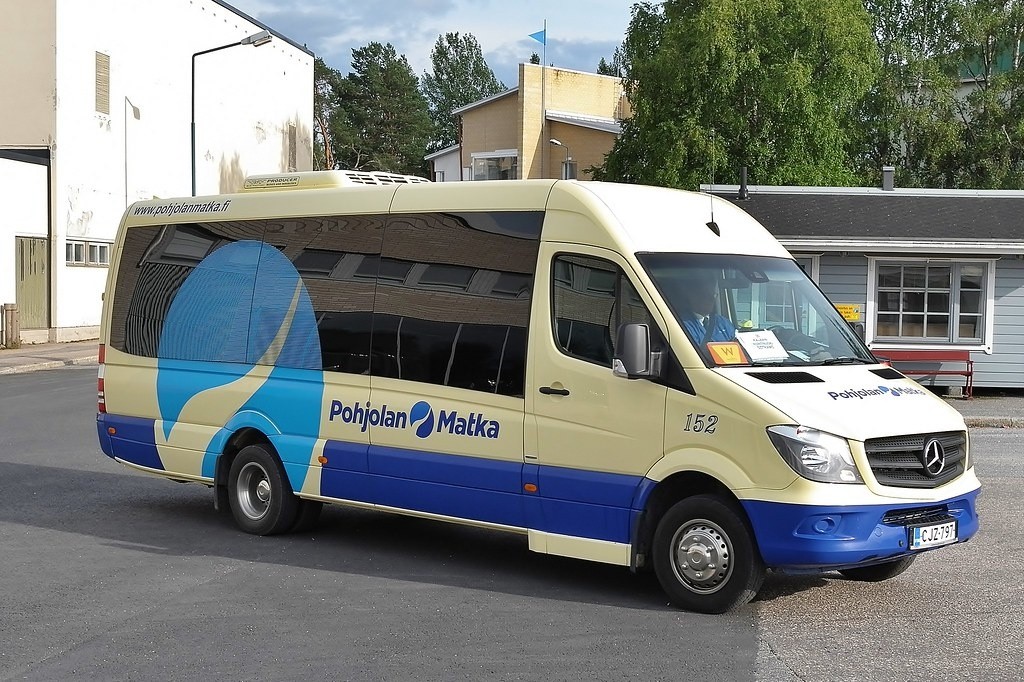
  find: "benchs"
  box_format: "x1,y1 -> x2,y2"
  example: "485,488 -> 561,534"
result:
869,348 -> 975,400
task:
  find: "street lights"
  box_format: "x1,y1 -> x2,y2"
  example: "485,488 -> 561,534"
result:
190,27 -> 272,199
550,139 -> 570,181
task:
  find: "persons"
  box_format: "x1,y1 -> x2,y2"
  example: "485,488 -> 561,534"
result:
676,280 -> 735,347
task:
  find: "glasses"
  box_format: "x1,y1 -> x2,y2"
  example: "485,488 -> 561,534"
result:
709,291 -> 719,298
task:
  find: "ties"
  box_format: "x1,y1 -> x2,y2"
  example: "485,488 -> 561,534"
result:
703,317 -> 710,331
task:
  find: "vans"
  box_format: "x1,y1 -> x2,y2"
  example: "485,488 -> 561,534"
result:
97,169 -> 983,613
